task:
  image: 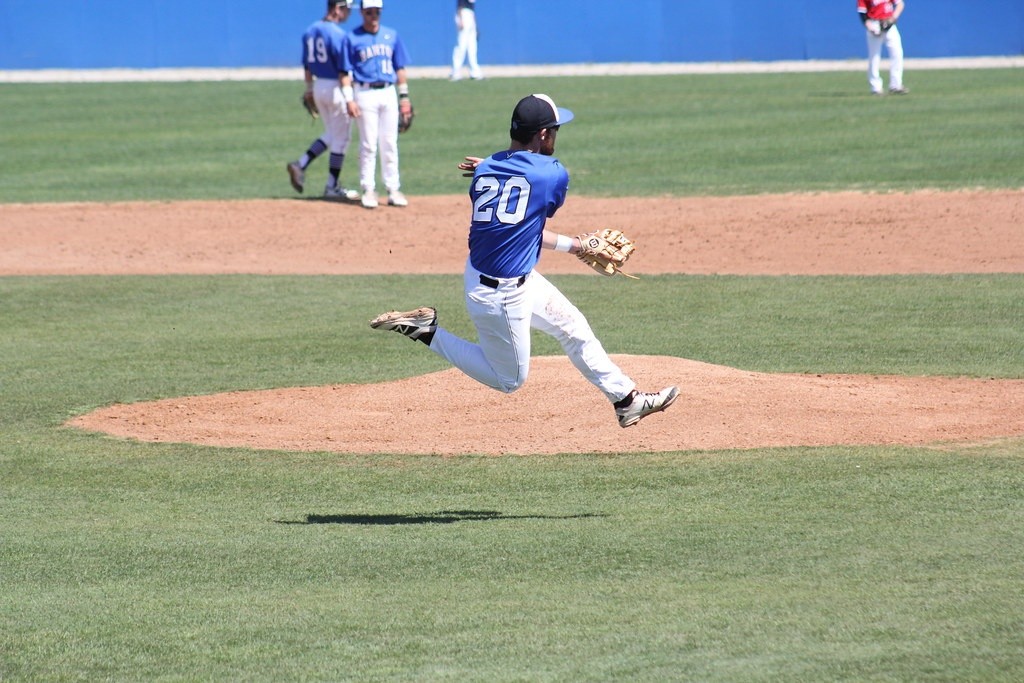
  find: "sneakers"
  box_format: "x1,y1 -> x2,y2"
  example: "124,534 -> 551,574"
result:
615,386 -> 680,428
369,307 -> 438,342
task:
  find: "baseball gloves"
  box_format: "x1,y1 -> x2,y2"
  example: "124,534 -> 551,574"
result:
302,92 -> 320,128
574,227 -> 642,281
880,18 -> 893,32
398,102 -> 415,134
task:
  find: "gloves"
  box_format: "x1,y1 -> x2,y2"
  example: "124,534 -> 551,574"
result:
397,94 -> 413,134
303,90 -> 319,119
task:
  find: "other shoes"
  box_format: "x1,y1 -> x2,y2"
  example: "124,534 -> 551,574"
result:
889,88 -> 909,95
288,161 -> 304,194
470,71 -> 482,80
389,191 -> 407,207
449,69 -> 461,81
322,186 -> 358,199
872,90 -> 884,96
360,190 -> 378,208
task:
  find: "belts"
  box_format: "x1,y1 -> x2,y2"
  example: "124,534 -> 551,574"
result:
478,274 -> 525,290
360,81 -> 394,89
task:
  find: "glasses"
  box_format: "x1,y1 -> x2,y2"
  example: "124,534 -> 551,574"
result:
549,124 -> 562,131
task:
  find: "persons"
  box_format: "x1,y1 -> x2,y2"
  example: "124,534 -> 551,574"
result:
287,0 -> 359,198
338,0 -> 414,209
371,93 -> 681,428
450,1 -> 485,81
857,0 -> 910,94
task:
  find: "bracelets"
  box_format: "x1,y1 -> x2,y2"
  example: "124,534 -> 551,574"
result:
556,235 -> 572,252
400,94 -> 408,98
342,87 -> 353,101
305,79 -> 313,93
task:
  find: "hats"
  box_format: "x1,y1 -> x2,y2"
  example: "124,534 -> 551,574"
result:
328,0 -> 360,9
361,0 -> 383,9
511,93 -> 573,132
